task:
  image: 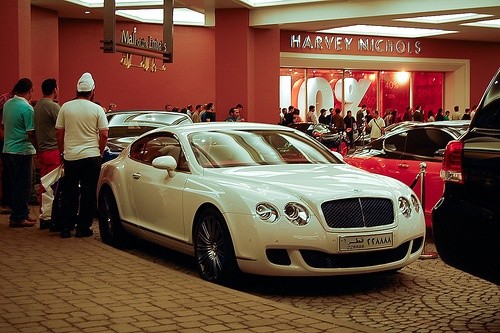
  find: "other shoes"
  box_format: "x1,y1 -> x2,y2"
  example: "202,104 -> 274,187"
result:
9,220 -> 34,227
27,217 -> 37,222
60,231 -> 71,238
75,229 -> 93,237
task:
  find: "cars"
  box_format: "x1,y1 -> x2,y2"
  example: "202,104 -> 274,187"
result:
271,122 -> 350,158
96,121 -> 427,287
345,119 -> 474,229
102,111 -> 194,164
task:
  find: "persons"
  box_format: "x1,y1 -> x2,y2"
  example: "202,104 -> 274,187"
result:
3,78 -> 38,227
55,72 -> 108,237
107,103 -> 116,113
279,105 -> 476,143
0,93 -> 11,215
164,102 -> 245,122
33,78 -> 64,229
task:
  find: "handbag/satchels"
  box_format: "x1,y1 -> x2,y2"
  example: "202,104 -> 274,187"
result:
39,164 -> 81,233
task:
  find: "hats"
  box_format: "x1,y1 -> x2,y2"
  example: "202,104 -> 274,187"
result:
77,72 -> 94,92
320,109 -> 327,113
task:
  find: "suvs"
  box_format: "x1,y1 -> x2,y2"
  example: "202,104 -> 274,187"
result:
433,70 -> 500,284
